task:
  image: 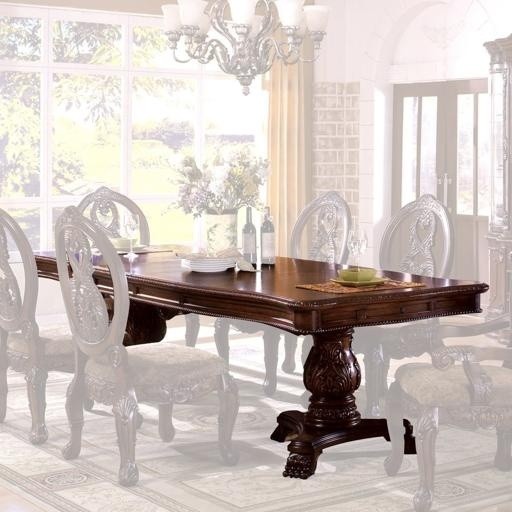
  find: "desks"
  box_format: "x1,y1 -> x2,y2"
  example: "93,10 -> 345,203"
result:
34,244 -> 490,480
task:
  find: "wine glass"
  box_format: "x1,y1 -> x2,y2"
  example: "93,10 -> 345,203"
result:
347,229 -> 368,271
123,214 -> 140,259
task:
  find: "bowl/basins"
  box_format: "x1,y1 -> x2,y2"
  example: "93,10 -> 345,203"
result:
337,267 -> 377,282
110,238 -> 138,248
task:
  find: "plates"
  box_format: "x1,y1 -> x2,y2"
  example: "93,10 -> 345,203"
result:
329,277 -> 389,288
113,244 -> 148,252
180,256 -> 236,273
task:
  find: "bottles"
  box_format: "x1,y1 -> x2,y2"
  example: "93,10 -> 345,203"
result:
241,206 -> 257,272
259,205 -> 276,269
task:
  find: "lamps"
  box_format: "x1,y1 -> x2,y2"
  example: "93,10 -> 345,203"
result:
157,0 -> 333,98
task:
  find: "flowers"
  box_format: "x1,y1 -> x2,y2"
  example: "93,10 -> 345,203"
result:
168,153 -> 272,218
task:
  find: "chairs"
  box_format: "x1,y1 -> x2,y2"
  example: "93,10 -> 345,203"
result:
56,207 -> 240,488
300,192 -> 454,416
185,184 -> 353,397
2,208 -> 144,447
77,188 -> 200,348
381,207 -> 509,508
